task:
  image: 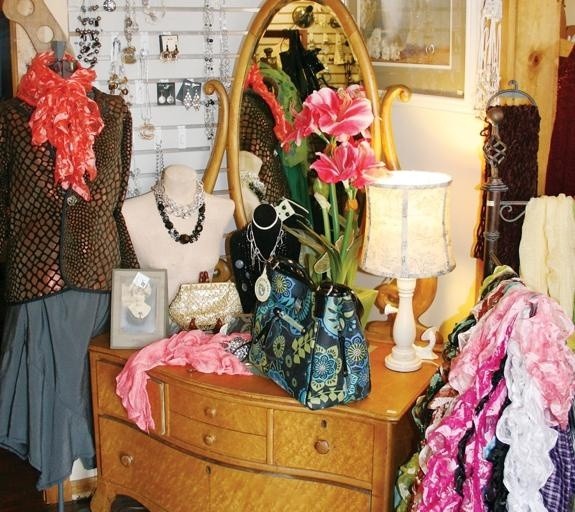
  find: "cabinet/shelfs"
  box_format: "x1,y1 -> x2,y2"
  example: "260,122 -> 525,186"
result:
87,329 -> 449,511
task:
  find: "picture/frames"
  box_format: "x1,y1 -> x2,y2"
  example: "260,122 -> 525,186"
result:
110,268 -> 168,348
347,1 -> 466,99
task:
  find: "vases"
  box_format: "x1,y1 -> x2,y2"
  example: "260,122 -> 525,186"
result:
353,290 -> 379,334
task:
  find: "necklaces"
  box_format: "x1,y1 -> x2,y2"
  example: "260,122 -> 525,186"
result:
151,180 -> 207,245
245,222 -> 283,301
137,51 -> 156,142
203,0 -> 234,144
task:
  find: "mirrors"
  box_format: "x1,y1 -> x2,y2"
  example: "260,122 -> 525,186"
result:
204,1 -> 444,350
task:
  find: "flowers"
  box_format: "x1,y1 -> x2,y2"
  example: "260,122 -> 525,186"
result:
293,86 -> 386,288
242,62 -> 314,229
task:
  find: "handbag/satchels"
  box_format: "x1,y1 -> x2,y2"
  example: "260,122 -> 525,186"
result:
247,256 -> 371,411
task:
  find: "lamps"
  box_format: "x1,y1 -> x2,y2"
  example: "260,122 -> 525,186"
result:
359,171 -> 456,372
